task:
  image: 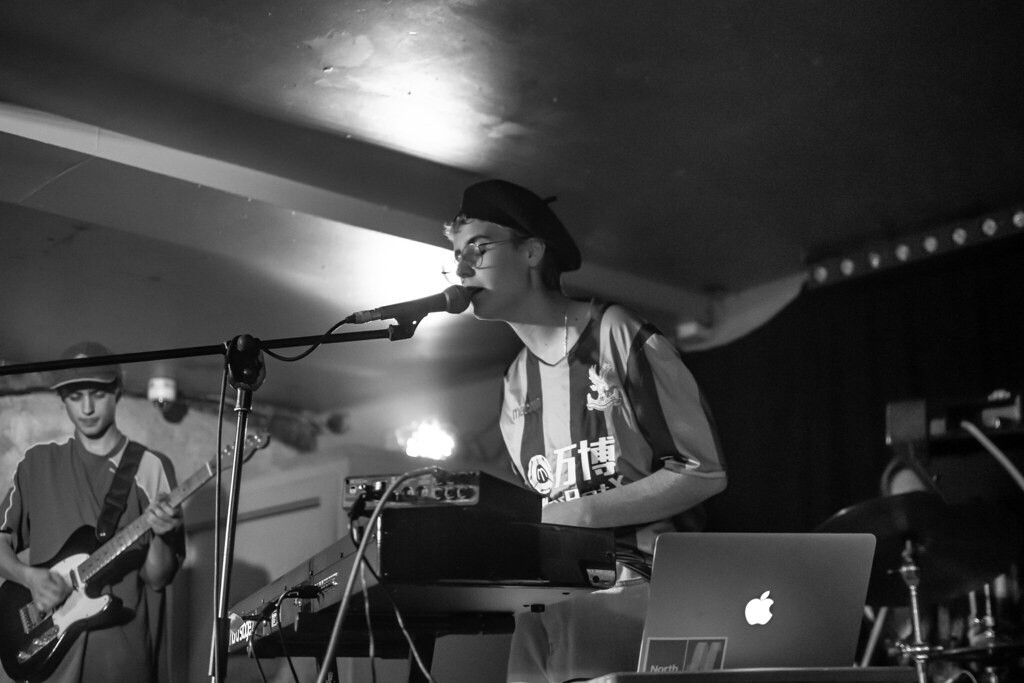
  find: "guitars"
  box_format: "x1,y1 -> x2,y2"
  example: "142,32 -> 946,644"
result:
0,427 -> 272,683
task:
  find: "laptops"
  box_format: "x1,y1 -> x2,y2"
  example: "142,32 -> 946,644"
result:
639,532 -> 877,673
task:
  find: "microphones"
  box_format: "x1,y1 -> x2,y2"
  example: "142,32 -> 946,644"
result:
346,285 -> 471,325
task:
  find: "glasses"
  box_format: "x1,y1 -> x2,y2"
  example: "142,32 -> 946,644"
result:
452,234 -> 534,270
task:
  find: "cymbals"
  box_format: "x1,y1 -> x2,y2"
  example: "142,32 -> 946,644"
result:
812,489 -> 1012,607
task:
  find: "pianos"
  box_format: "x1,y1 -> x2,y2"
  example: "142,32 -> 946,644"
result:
225,505 -> 617,658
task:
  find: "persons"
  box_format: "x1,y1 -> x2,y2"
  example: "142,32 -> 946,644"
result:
0,342 -> 187,683
444,178 -> 730,683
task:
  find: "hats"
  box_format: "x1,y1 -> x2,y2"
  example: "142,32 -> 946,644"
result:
456,179 -> 582,272
51,341 -> 121,391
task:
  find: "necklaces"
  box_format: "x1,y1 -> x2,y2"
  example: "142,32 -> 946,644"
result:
563,297 -> 571,354
